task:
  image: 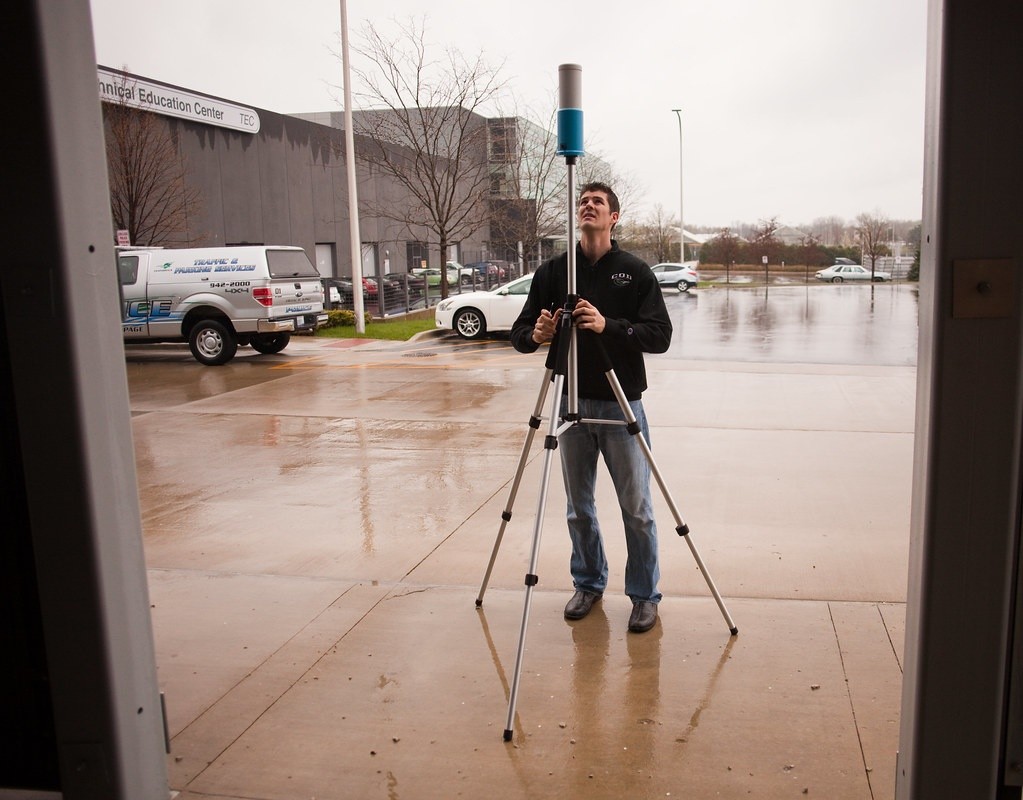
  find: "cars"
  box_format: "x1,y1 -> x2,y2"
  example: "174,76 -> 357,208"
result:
815,264 -> 893,285
650,263 -> 699,293
320,260 -> 521,307
436,273 -> 537,340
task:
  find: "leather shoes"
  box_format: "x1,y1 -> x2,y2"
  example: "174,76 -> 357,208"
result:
565,591 -> 602,620
627,602 -> 659,630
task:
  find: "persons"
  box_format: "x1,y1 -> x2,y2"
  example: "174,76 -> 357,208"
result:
510,181 -> 675,633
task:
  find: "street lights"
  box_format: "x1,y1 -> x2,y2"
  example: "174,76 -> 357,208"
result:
672,110 -> 685,264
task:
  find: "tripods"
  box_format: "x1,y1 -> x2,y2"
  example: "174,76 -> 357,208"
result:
468,155 -> 743,742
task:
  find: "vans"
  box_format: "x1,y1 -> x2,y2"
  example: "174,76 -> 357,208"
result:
113,245 -> 324,367
